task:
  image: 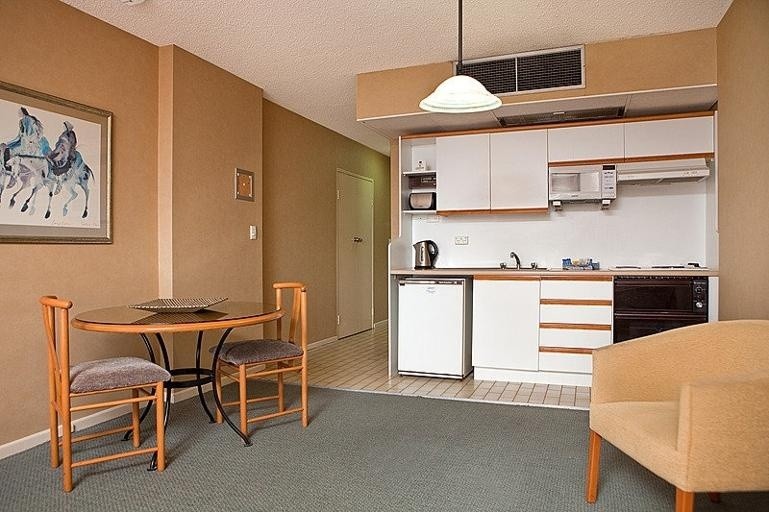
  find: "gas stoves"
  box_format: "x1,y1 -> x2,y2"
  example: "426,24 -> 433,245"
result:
607,261 -> 710,272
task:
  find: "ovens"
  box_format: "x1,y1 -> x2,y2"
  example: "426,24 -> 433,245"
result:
613,275 -> 708,347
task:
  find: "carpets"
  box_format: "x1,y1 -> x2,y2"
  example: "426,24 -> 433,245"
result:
1,376 -> 768,509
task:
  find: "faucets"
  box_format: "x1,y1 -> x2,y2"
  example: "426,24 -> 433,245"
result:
509,252 -> 520,270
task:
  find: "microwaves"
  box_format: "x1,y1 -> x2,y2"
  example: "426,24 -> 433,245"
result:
548,164 -> 617,210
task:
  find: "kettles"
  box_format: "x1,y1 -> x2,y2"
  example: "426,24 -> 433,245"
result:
412,240 -> 439,270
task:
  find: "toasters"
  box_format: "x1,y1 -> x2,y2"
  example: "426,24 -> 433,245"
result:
408,192 -> 436,210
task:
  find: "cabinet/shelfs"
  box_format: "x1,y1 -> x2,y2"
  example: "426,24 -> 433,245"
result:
471,276 -> 540,372
399,136 -> 437,214
540,275 -> 617,354
433,127 -> 550,212
547,108 -> 719,162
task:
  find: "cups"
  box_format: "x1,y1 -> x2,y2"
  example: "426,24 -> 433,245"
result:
562,258 -> 571,270
415,160 -> 427,171
592,263 -> 599,270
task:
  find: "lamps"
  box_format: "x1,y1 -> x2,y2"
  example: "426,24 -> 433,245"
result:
416,0 -> 503,115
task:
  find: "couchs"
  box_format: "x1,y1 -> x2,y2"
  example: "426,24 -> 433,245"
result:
584,318 -> 769,511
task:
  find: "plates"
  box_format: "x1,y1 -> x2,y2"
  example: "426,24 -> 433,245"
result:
129,297 -> 228,314
131,309 -> 227,325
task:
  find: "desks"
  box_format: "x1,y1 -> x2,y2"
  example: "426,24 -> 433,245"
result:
70,299 -> 286,473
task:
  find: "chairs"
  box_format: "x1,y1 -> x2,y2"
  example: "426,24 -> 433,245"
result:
209,279 -> 318,433
40,293 -> 168,493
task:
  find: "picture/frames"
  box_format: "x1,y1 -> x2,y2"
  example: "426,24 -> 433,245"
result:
234,166 -> 256,203
1,79 -> 114,245
